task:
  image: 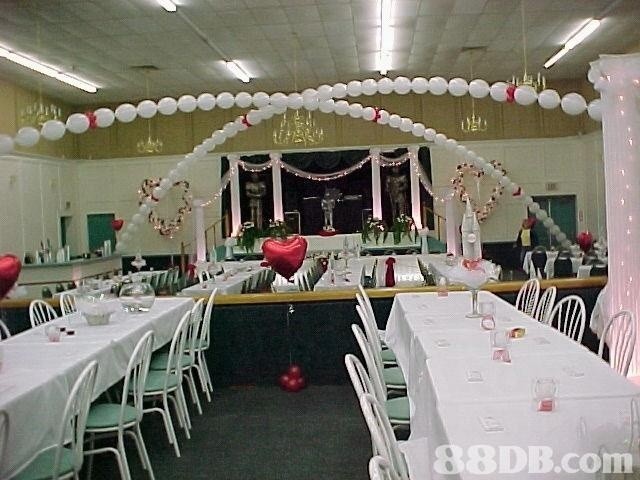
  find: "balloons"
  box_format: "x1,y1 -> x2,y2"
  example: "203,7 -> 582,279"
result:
0,75 -> 604,253
262,235 -> 307,278
0,253 -> 22,300
278,367 -> 308,394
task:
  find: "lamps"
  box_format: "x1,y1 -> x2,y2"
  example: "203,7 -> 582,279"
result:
155,0 -> 178,15
226,61 -> 250,83
20,0 -> 62,126
543,18 -> 600,69
131,65 -> 164,154
505,0 -> 546,103
379,0 -> 390,78
0,45 -> 98,99
273,34 -> 324,148
461,46 -> 488,134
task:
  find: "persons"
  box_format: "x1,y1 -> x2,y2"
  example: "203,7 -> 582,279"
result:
516,218 -> 538,276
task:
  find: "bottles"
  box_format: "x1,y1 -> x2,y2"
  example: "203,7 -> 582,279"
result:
63,245 -> 69,262
104,240 -> 112,256
